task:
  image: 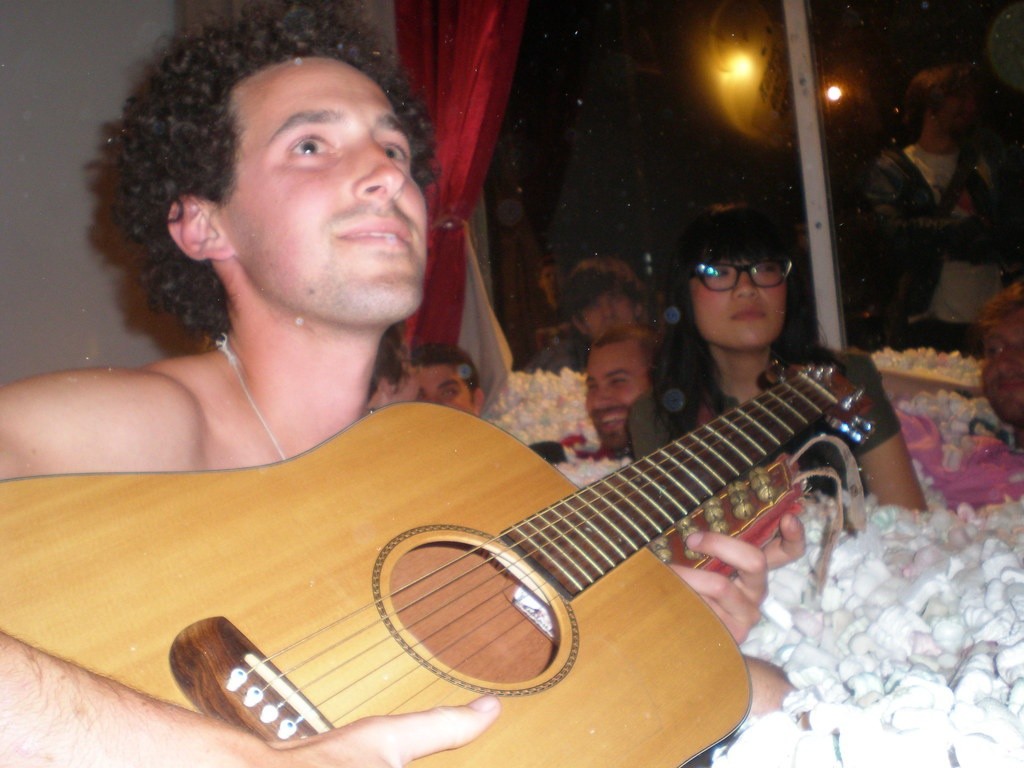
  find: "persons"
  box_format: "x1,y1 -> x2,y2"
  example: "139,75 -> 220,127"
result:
2,0 -> 1024,767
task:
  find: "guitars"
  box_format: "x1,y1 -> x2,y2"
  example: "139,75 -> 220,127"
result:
1,357 -> 884,768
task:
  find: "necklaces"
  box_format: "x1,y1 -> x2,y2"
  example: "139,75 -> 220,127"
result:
214,332 -> 285,460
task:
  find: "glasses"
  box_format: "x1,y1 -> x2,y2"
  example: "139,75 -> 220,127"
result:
690,258 -> 793,292
